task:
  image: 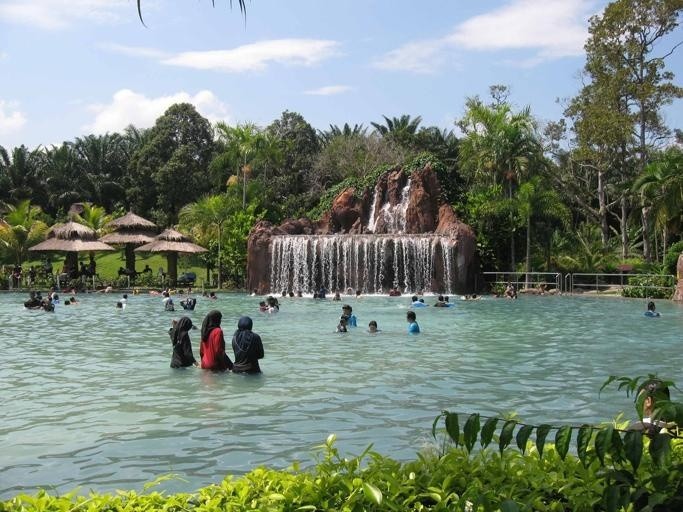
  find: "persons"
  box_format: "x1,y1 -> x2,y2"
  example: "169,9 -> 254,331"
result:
65,297 -> 79,305
105,283 -> 112,293
5,255 -> 96,289
281,284 -> 361,300
504,281 -> 518,301
406,311 -> 420,334
644,300 -> 660,316
202,291 -> 217,300
251,288 -> 257,296
260,296 -> 279,314
25,287 -> 59,312
116,295 -> 128,310
197,310 -> 234,373
367,321 -> 381,332
167,314 -> 199,372
465,294 -> 480,301
337,304 -> 356,333
231,316 -> 265,374
410,296 -> 455,308
119,265 -> 152,283
149,287 -> 197,311
390,288 -> 401,296
